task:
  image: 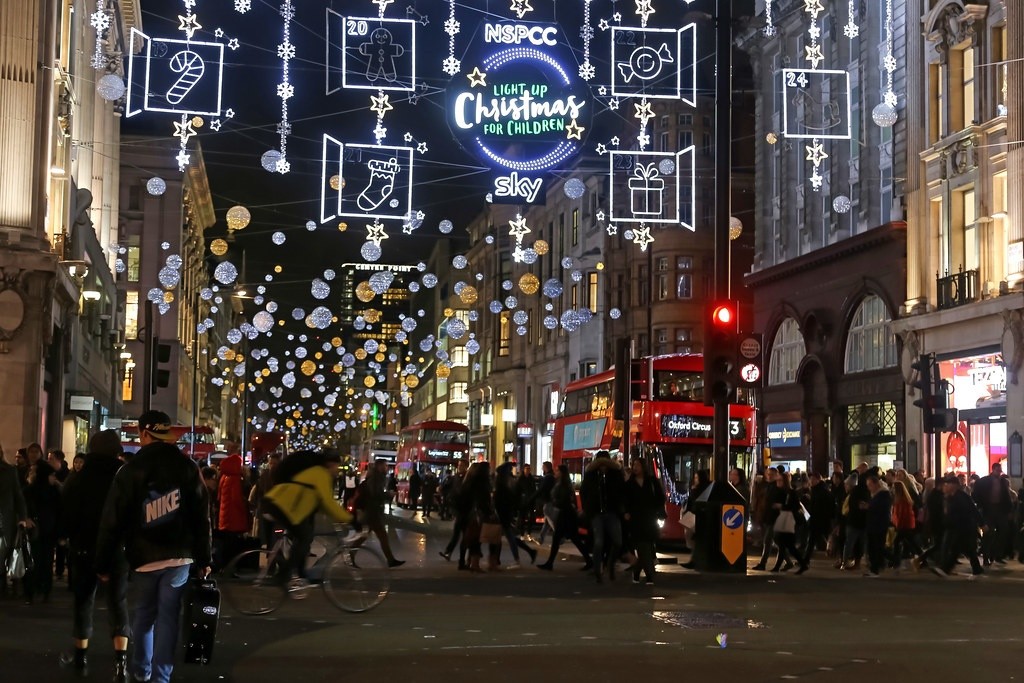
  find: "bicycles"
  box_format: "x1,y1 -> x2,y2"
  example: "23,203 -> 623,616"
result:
221,514 -> 391,615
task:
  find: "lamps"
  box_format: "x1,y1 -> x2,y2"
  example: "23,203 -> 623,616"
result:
67,265 -> 135,388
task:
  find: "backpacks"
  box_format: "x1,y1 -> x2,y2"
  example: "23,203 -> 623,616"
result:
273,451 -> 325,489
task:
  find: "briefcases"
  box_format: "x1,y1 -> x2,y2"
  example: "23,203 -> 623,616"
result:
182,575 -> 220,665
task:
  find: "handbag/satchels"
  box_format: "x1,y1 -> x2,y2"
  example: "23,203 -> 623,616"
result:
773,510 -> 795,533
885,526 -> 898,547
479,521 -> 501,544
6,525 -> 26,579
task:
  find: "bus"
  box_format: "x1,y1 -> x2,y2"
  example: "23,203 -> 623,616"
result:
395,419 -> 471,509
121,420 -> 218,461
552,354 -> 771,538
359,436 -> 399,477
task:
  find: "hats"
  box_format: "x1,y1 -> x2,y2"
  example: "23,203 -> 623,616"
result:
139,409 -> 176,440
843,472 -> 859,486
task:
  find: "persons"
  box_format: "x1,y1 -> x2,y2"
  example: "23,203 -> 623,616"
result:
99,410 -> 213,683
199,444 -> 406,592
730,460 -> 1024,579
682,470 -> 708,568
409,451 -> 664,585
0,428 -> 135,683
667,382 -> 681,399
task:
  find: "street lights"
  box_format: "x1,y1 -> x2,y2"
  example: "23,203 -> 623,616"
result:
190,290 -> 246,458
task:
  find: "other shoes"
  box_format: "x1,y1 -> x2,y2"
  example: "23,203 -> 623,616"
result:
439,552 -> 451,561
633,569 -> 641,583
680,560 -> 696,569
111,650 -> 130,683
536,562 -> 554,571
578,555 -> 620,585
75,656 -> 87,677
752,553 -> 1024,580
530,549 -> 537,564
348,551 -> 359,567
645,574 -> 655,585
457,552 -> 521,573
388,558 -> 405,567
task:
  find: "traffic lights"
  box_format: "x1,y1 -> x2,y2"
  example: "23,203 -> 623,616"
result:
911,355 -> 957,435
700,299 -> 742,406
152,336 -> 171,394
